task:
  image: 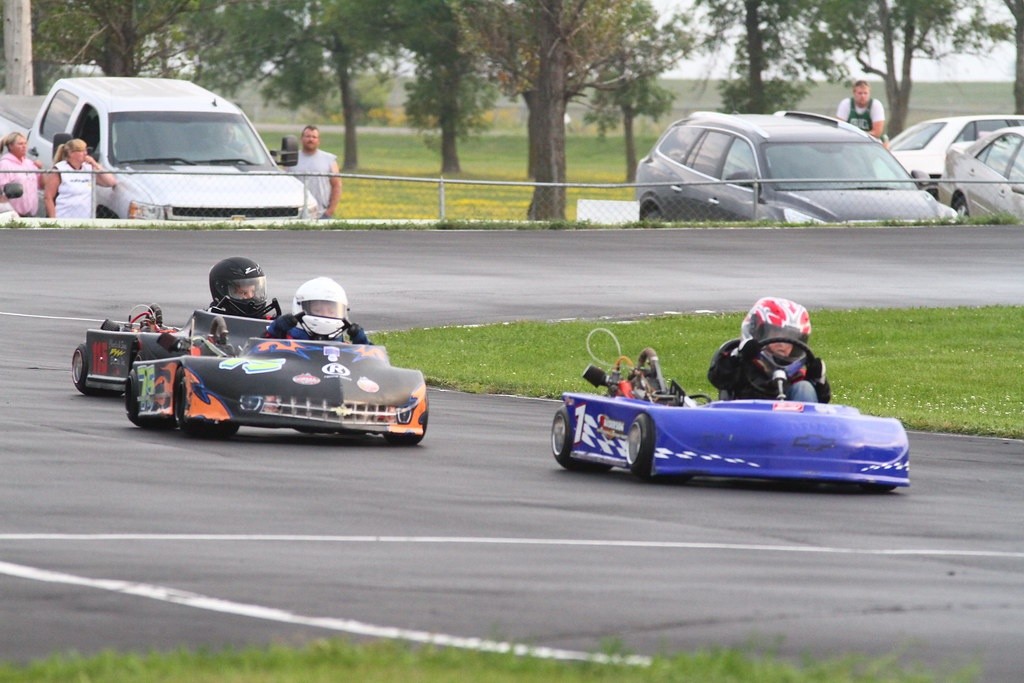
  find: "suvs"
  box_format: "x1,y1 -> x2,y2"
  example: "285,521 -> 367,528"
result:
635,109 -> 958,227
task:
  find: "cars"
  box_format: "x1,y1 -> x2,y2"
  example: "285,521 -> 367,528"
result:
940,125 -> 1023,224
27,75 -> 320,224
885,115 -> 1024,198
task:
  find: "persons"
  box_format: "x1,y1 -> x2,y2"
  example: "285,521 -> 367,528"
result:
44,137 -> 117,220
707,297 -> 833,406
204,257 -> 281,321
262,276 -> 375,345
0,131 -> 46,216
836,80 -> 890,150
281,126 -> 341,217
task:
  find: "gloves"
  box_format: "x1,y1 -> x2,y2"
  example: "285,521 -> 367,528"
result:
347,322 -> 371,345
205,304 -> 225,314
808,358 -> 826,393
730,337 -> 762,366
266,312 -> 297,338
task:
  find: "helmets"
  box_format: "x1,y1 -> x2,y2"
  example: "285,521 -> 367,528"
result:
739,297 -> 812,363
209,257 -> 269,315
295,278 -> 346,335
